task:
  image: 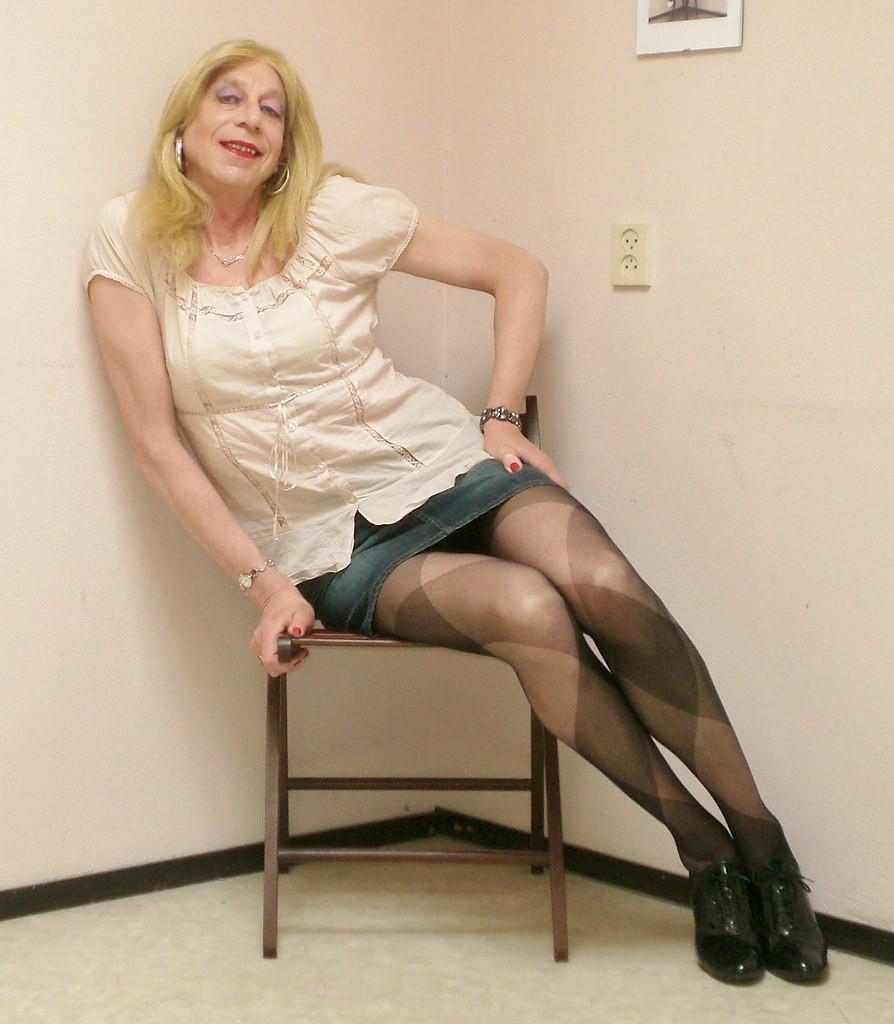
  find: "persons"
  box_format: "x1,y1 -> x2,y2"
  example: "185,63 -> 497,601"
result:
84,38 -> 830,989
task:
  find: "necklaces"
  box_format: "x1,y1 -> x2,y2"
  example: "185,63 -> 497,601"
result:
200,236 -> 249,267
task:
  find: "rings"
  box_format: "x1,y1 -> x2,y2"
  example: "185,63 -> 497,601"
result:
257,655 -> 263,664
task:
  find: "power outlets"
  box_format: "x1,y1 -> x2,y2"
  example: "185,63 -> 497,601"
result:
610,221 -> 655,286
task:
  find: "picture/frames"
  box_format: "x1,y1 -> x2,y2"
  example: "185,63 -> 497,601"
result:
637,0 -> 744,56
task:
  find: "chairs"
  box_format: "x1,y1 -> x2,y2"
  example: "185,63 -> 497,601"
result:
261,395 -> 568,963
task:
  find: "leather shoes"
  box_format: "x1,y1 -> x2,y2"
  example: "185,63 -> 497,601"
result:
689,857 -> 828,982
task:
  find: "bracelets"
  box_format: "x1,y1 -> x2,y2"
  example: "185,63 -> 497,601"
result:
239,558 -> 274,591
480,405 -> 522,434
